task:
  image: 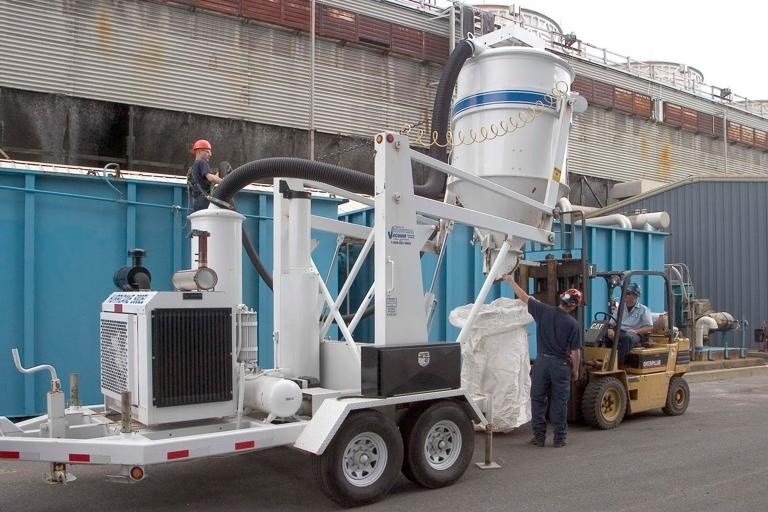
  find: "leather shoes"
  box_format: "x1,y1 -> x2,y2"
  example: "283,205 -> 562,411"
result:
530,438 -> 545,447
552,440 -> 570,448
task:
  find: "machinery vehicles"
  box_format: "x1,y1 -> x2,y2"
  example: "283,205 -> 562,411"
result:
515,252 -> 690,429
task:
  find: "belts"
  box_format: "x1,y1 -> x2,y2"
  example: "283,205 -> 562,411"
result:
543,352 -> 562,360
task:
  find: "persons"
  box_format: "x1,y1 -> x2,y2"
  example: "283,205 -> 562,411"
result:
606,280 -> 656,371
501,273 -> 583,450
187,138 -> 224,211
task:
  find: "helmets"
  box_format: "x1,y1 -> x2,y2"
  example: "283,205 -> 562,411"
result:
626,282 -> 641,297
191,139 -> 212,154
559,288 -> 584,308
